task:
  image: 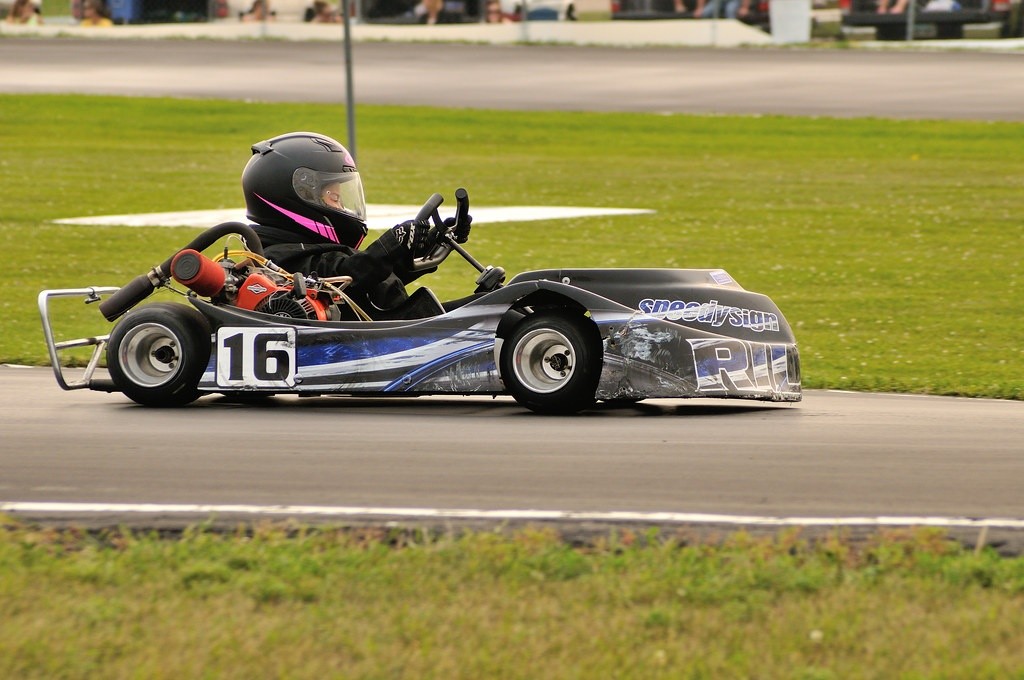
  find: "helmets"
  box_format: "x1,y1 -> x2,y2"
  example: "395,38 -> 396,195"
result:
243,131 -> 370,250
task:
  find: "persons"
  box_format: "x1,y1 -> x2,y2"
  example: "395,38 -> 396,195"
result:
0,0 -> 44,24
477,1 -> 513,23
675,0 -> 750,19
76,0 -> 114,26
241,1 -> 343,24
241,132 -> 473,321
418,0 -> 460,24
875,0 -> 909,16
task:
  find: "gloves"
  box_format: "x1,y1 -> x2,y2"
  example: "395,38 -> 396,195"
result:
390,220 -> 431,253
431,216 -> 472,245
